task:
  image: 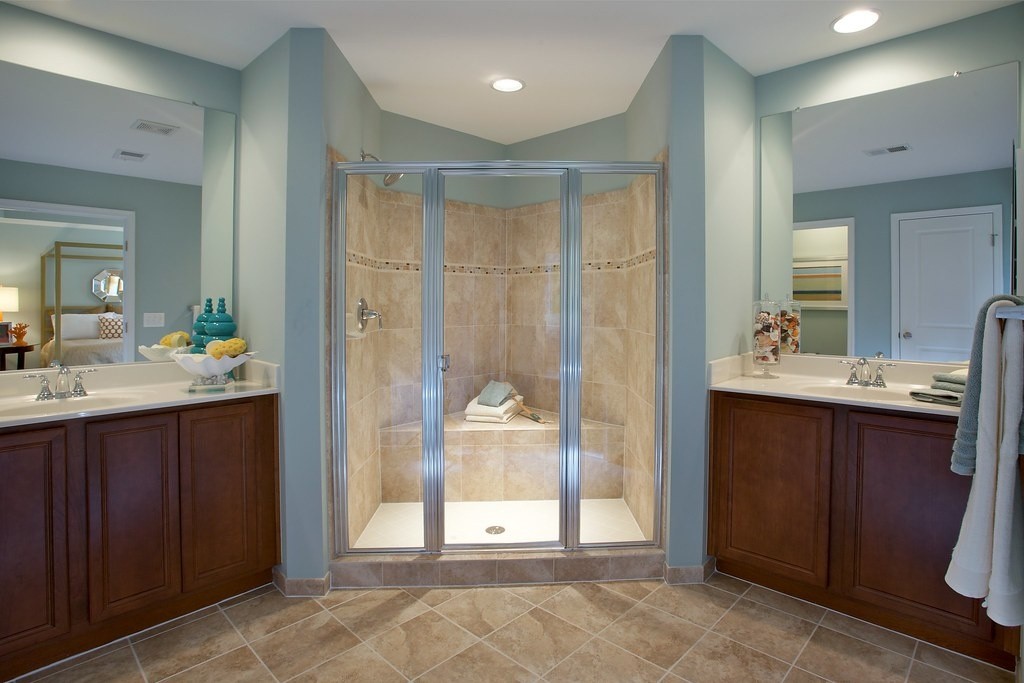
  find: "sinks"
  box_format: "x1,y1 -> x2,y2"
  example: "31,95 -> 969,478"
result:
0,397 -> 139,417
800,385 -> 910,402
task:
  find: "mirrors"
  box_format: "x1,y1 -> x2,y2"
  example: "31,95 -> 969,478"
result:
91,268 -> 124,302
0,60 -> 237,370
760,59 -> 1021,366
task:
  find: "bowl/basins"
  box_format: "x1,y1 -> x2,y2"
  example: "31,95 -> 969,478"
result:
138,343 -> 180,363
170,346 -> 258,378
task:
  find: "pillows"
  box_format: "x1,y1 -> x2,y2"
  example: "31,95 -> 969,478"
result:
98,315 -> 124,340
51,312 -> 114,341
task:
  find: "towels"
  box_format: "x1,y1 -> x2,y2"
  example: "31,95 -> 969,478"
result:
943,294 -> 1024,627
464,379 -> 524,424
910,368 -> 969,408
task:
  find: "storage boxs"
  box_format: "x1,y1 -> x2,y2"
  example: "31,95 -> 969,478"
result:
0,322 -> 13,346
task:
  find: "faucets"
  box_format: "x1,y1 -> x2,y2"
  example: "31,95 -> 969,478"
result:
53,367 -> 72,399
47,360 -> 61,368
873,351 -> 884,358
857,358 -> 872,386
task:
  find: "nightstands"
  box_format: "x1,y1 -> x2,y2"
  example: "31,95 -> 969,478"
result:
0,343 -> 39,372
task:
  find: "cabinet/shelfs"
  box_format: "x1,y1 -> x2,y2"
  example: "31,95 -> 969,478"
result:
0,391 -> 283,683
706,390 -> 1024,674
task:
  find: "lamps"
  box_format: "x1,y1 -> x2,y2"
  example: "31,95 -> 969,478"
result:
0,285 -> 19,322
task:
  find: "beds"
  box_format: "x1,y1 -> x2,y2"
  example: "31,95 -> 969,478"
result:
39,241 -> 124,367
